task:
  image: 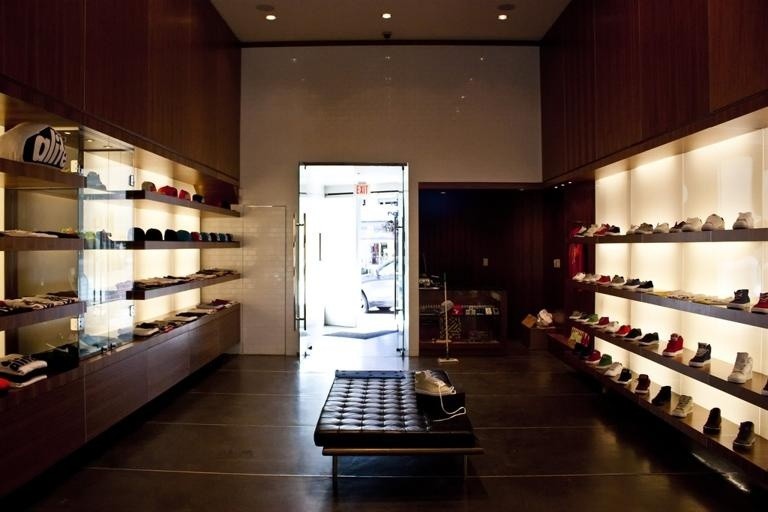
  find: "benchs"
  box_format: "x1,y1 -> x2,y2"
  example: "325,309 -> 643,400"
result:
314,369 -> 486,500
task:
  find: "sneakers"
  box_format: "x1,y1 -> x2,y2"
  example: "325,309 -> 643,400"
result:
733,212 -> 754,229
701,213 -> 725,230
728,352 -> 754,384
572,272 -> 654,292
671,395 -> 693,417
652,386 -> 671,406
415,371 -> 452,396
733,421 -> 755,449
752,292 -> 768,313
575,223 -> 620,238
638,332 -> 659,346
728,289 -> 750,310
689,342 -> 711,367
626,217 -> 702,234
663,334 -> 684,356
569,311 -> 643,341
585,349 -> 633,384
635,374 -> 651,394
703,408 -> 721,435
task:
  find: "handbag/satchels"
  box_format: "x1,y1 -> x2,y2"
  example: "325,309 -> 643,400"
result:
0,121 -> 67,169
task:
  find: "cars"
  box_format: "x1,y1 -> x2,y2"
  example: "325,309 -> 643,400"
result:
360,272 -> 396,315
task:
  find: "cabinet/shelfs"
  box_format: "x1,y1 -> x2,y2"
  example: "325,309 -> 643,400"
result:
546,228 -> 768,488
